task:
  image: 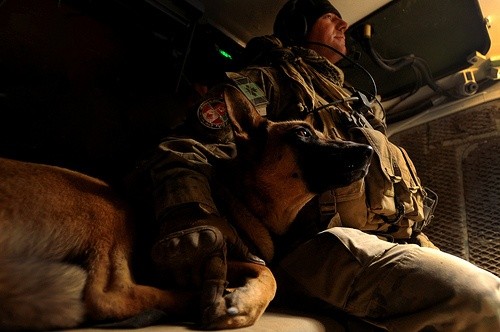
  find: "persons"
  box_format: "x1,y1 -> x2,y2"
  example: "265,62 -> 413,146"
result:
139,0 -> 500,332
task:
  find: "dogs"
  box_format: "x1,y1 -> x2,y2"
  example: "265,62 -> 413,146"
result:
0,84 -> 375,332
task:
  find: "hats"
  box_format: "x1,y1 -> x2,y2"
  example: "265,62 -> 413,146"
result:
274,0 -> 341,48
